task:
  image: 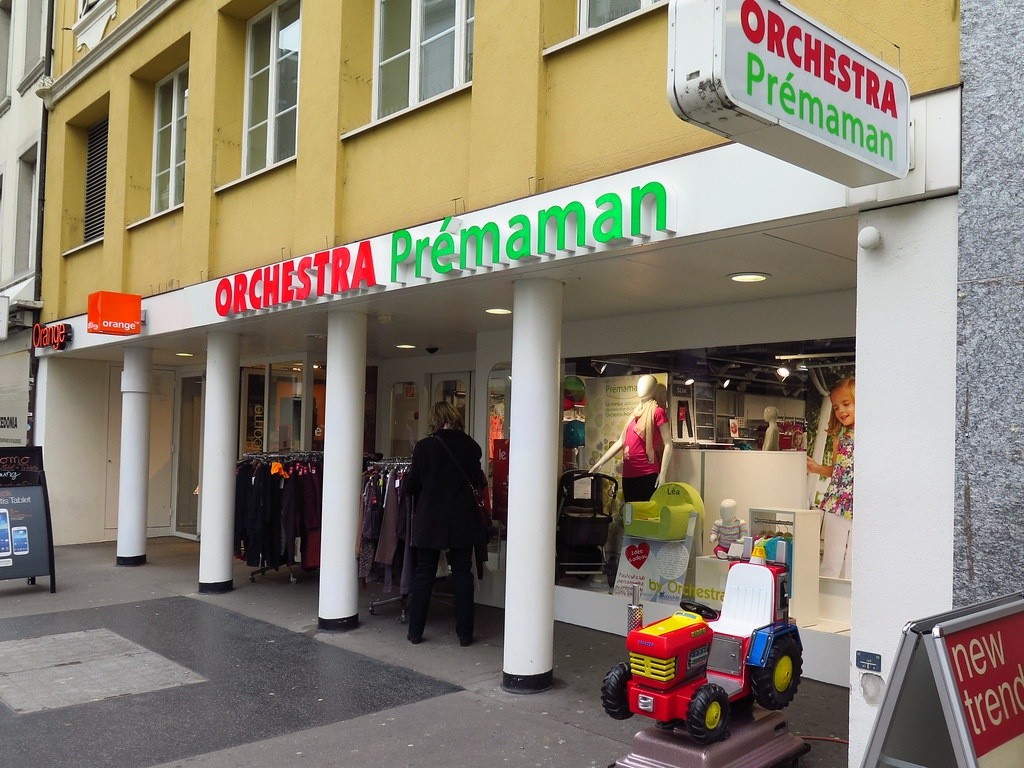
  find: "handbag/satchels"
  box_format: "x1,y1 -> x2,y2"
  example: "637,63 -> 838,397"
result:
475,502 -> 492,528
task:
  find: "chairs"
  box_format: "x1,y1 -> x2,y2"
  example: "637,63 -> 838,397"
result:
706,564 -> 774,637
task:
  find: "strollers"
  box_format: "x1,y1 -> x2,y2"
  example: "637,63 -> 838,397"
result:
554,469 -> 620,586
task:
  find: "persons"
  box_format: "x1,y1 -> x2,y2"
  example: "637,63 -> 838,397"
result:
588,375 -> 675,502
762,406 -> 779,452
710,499 -> 748,559
808,376 -> 854,580
399,402 -> 488,647
793,431 -> 803,447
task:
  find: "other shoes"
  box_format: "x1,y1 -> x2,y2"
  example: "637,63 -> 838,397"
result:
460,636 -> 475,647
407,633 -> 422,644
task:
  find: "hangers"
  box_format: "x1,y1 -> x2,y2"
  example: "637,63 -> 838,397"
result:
245,449 -> 322,464
367,456 -> 412,472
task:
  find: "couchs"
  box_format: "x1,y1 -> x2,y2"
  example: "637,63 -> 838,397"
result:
623,482 -> 704,541
